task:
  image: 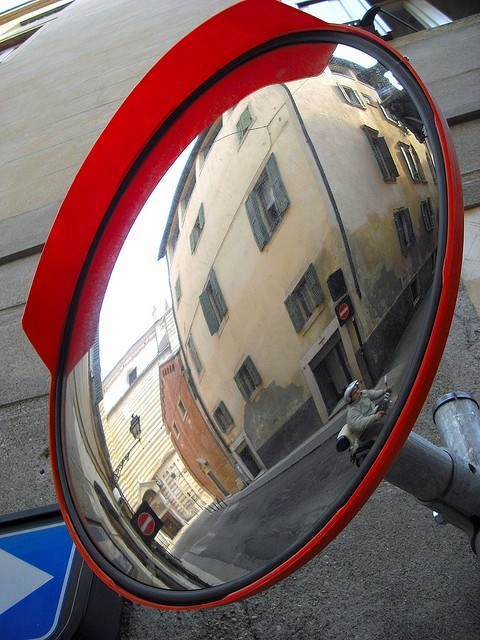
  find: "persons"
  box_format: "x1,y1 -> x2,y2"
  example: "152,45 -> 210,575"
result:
345,379 -> 392,442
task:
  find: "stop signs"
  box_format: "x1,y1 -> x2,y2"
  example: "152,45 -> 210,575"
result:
129,500 -> 163,547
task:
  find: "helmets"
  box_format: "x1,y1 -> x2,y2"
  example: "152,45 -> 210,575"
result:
344,380 -> 360,405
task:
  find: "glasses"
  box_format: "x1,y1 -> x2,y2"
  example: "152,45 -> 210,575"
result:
352,388 -> 358,395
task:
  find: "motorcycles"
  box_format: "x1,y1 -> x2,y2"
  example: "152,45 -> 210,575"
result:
336,375 -> 396,468
